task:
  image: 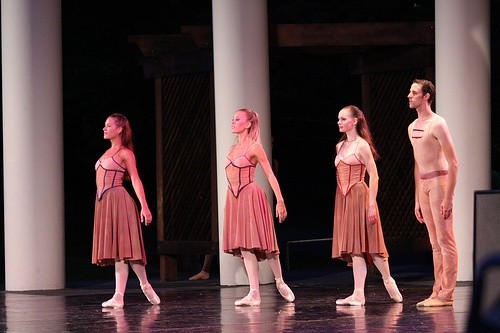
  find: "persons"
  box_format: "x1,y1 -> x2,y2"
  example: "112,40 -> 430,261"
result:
407,79 -> 458,307
332,105 -> 403,306
189,253 -> 213,280
91,112 -> 160,308
223,108 -> 295,306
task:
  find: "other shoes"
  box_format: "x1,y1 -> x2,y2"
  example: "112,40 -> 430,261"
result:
234,289 -> 261,306
382,276 -> 403,303
140,283 -> 161,304
416,297 -> 435,307
423,298 -> 453,307
274,277 -> 295,302
102,291 -> 124,309
189,271 -> 209,280
336,289 -> 366,306
